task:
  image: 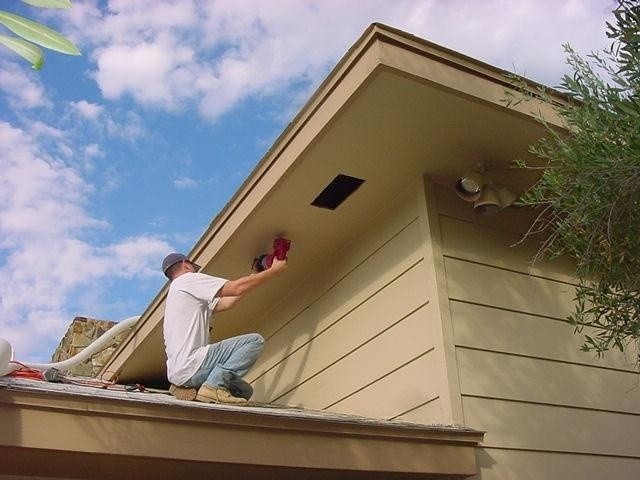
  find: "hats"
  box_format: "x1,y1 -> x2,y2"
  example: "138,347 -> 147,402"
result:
163,253 -> 201,274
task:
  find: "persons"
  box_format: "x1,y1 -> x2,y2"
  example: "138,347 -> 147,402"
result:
161,252 -> 289,406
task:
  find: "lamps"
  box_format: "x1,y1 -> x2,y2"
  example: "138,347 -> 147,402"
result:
456,162 -> 518,218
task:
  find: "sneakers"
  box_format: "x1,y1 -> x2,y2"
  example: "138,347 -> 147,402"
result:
168,384 -> 197,401
195,384 -> 248,406
